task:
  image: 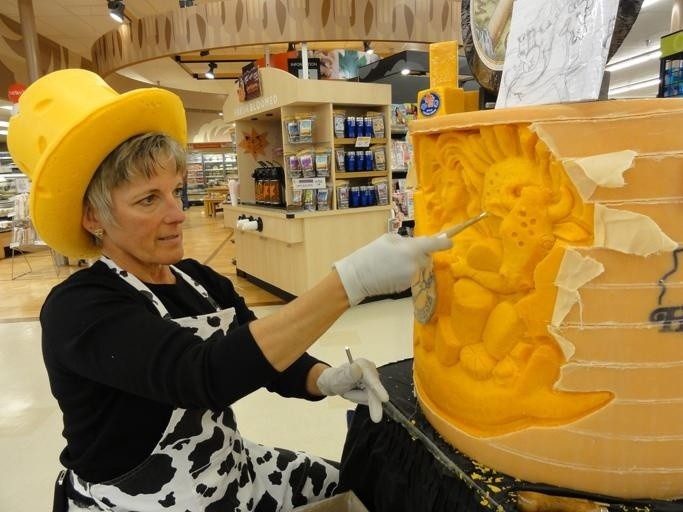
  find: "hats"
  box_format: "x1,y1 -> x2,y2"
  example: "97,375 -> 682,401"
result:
5,69 -> 187,261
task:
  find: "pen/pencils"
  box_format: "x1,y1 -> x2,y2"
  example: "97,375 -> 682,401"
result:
345,347 -> 360,390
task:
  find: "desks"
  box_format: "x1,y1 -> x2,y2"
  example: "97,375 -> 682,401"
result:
339,359 -> 683,512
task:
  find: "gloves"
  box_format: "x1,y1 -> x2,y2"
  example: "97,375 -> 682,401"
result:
316,357 -> 389,424
331,230 -> 454,308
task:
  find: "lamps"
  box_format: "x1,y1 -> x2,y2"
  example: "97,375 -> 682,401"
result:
107,0 -> 136,23
364,41 -> 373,55
288,42 -> 296,50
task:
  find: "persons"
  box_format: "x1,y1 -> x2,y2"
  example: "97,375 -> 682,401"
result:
40,128 -> 454,512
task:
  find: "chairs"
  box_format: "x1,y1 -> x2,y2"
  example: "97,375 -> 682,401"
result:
9,193 -> 60,281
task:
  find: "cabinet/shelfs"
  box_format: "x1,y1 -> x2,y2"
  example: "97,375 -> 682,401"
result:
185,67 -> 414,305
0,135 -> 32,259
